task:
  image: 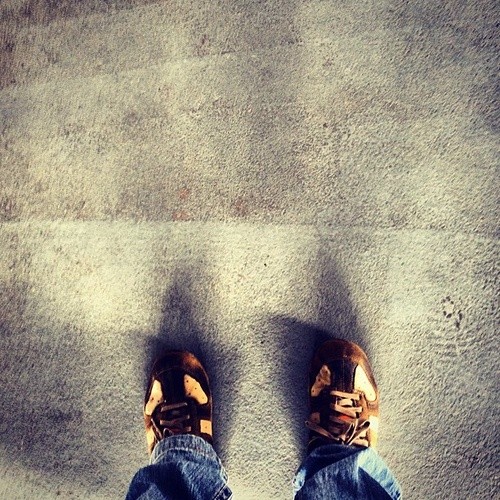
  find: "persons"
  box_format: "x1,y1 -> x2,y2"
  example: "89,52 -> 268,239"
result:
123,340 -> 403,500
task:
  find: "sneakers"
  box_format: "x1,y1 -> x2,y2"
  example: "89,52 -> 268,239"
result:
302,339 -> 379,470
143,350 -> 216,461
